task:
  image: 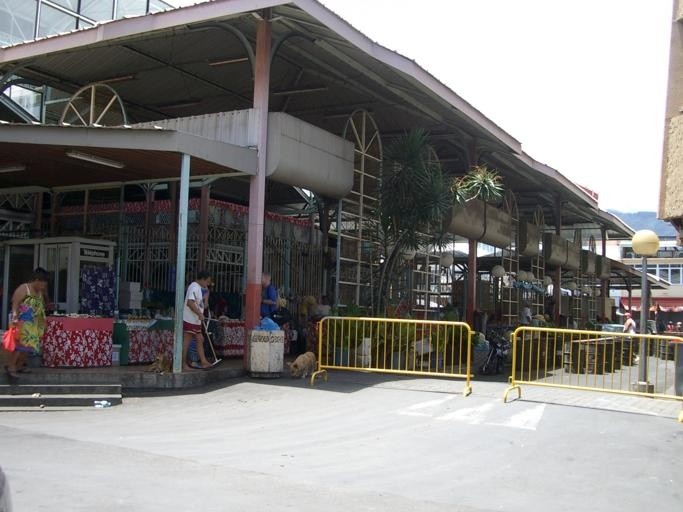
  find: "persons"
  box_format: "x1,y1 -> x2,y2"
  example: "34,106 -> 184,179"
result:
667,321 -> 674,332
623,312 -> 637,334
7,266 -> 51,377
261,271 -> 280,319
519,300 -> 533,324
183,270 -> 213,371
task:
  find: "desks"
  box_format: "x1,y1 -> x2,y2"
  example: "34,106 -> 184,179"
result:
39,316 -> 175,369
206,318 -> 295,360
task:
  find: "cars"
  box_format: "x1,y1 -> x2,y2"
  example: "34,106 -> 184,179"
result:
594,324 -> 623,337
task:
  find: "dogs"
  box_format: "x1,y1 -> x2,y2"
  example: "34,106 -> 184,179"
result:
142,351 -> 170,375
289,352 -> 317,378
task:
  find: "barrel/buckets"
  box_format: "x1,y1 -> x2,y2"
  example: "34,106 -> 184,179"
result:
564,338 -> 674,376
516,338 -> 556,370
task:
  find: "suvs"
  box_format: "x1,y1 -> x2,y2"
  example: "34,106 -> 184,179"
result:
632,319 -> 657,334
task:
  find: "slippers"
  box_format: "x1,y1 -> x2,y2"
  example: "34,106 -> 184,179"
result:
3,365 -> 21,379
16,366 -> 32,373
183,367 -> 196,372
200,365 -> 215,371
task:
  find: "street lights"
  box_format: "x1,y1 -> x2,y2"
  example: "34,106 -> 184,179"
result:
629,229 -> 660,399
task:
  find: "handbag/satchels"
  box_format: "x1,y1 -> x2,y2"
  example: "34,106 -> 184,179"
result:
270,307 -> 292,326
2,327 -> 17,353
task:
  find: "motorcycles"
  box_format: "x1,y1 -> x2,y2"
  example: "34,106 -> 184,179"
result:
478,336 -> 508,376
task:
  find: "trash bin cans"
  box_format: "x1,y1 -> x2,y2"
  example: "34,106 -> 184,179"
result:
250,317 -> 285,377
